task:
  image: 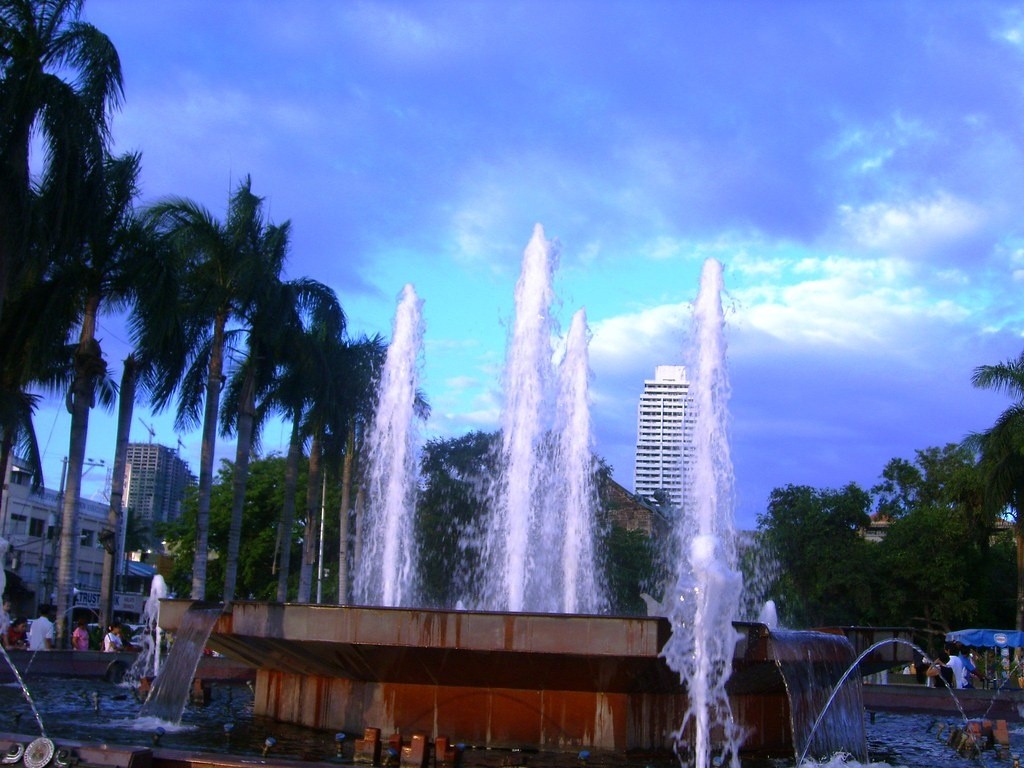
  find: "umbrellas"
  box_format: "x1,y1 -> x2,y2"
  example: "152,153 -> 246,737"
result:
945,629 -> 1024,647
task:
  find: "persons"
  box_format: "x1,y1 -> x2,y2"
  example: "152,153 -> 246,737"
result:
909,643 -> 1024,693
72,618 -> 89,653
100,621 -> 138,654
0,598 -> 54,653
